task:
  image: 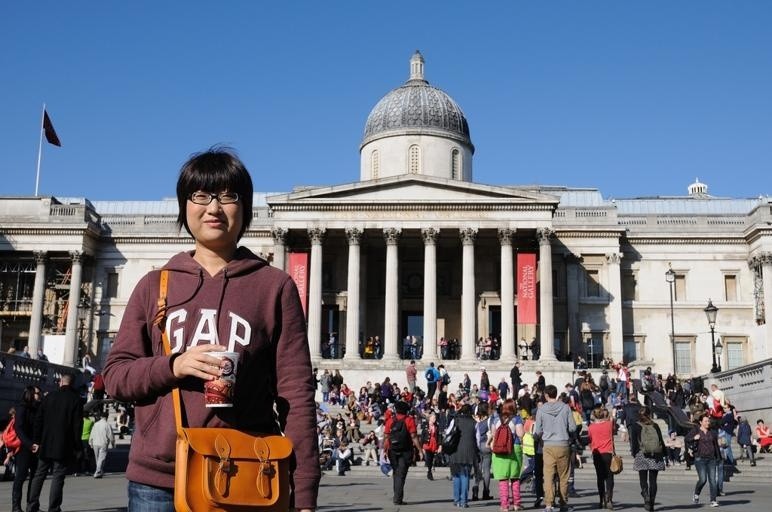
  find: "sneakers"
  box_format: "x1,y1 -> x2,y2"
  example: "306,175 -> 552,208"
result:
718,491 -> 725,496
709,500 -> 719,507
750,461 -> 756,465
453,501 -> 470,508
392,500 -> 408,505
534,492 -> 580,512
739,455 -> 744,463
692,493 -> 700,504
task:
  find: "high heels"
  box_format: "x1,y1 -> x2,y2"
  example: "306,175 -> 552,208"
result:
500,505 -> 509,511
513,504 -> 524,511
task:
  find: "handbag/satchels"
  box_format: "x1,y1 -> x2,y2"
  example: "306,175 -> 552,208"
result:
684,439 -> 699,452
443,373 -> 450,384
174,425 -> 294,511
609,454 -> 623,474
442,426 -> 461,455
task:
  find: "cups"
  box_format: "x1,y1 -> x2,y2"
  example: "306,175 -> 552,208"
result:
201,351 -> 240,408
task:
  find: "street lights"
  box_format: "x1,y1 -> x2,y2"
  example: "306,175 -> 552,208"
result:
702,297 -> 724,375
665,262 -> 678,379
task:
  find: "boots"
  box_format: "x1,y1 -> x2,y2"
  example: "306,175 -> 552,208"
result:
471,485 -> 478,500
482,488 -> 493,499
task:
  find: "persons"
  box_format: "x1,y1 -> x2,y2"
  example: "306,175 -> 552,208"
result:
365,335 -> 381,360
322,332 -> 336,359
0,371 -> 134,512
438,336 -> 459,360
37,348 -> 47,361
404,335 -> 418,359
477,336 -> 500,360
312,356 -> 772,511
99,141 -> 322,512
518,336 -> 538,360
20,345 -> 31,358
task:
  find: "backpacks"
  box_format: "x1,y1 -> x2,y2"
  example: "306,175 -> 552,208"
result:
425,367 -> 435,381
634,420 -> 664,454
492,413 -> 516,454
388,411 -> 413,455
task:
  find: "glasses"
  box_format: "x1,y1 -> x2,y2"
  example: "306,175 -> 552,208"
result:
33,390 -> 43,397
189,190 -> 240,205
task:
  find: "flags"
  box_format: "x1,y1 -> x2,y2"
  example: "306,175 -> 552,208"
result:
43,111 -> 63,147
